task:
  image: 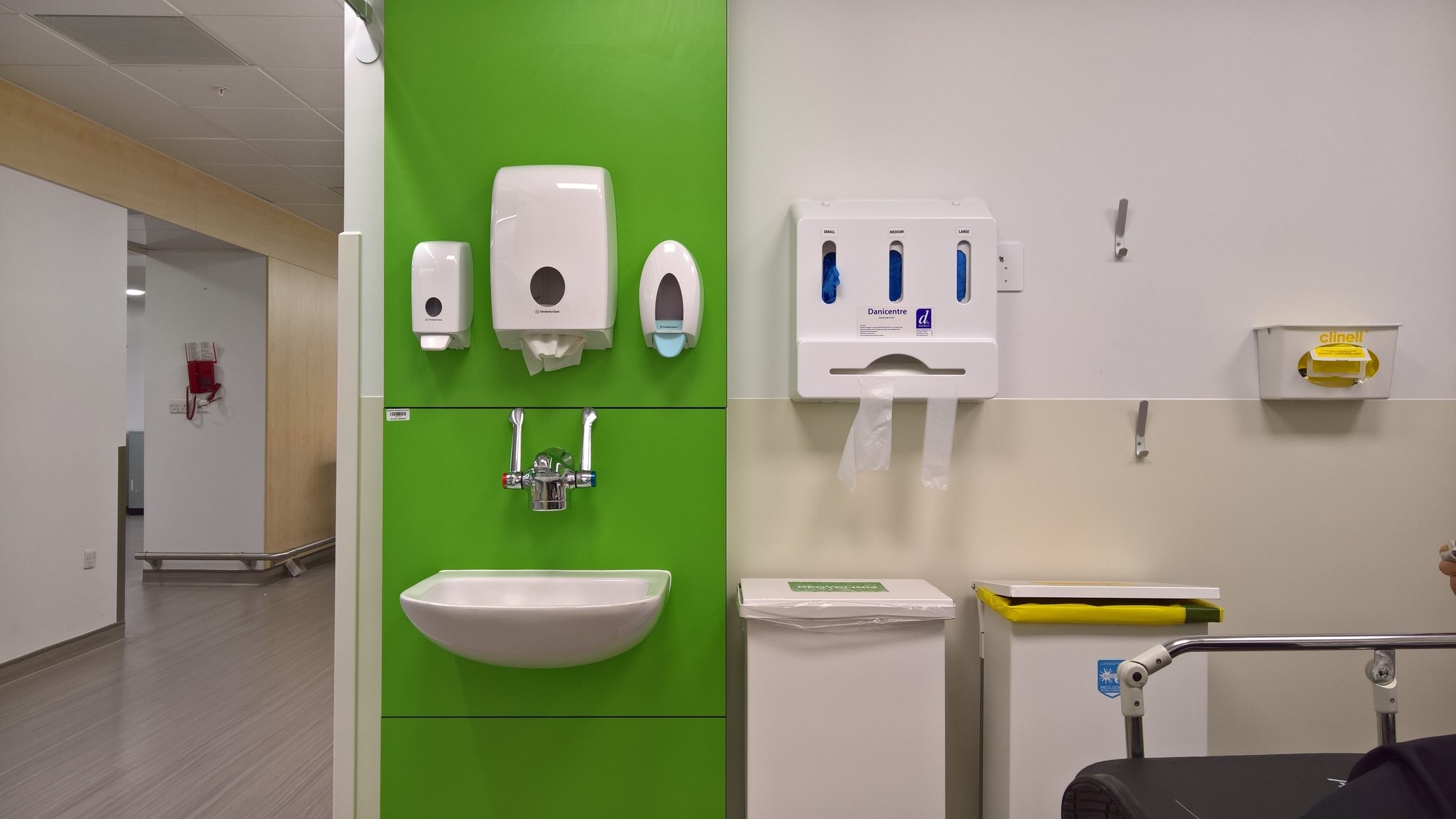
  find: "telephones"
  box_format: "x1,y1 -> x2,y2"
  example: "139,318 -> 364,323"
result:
188,360 -> 215,394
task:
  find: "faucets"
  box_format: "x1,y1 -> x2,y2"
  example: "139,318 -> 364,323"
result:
501,405 -> 598,513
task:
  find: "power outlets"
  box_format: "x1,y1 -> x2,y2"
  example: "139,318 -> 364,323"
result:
169,399 -> 192,415
83,550 -> 96,569
196,400 -> 211,413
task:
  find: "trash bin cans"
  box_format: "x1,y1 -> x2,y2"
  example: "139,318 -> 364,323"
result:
734,577 -> 956,819
971,577 -> 1223,819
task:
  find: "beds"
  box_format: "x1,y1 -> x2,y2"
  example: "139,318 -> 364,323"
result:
1059,633 -> 1456,819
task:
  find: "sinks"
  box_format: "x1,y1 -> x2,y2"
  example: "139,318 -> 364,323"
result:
398,569 -> 673,671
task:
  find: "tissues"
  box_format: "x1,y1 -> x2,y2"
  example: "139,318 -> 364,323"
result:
486,165 -> 619,377
